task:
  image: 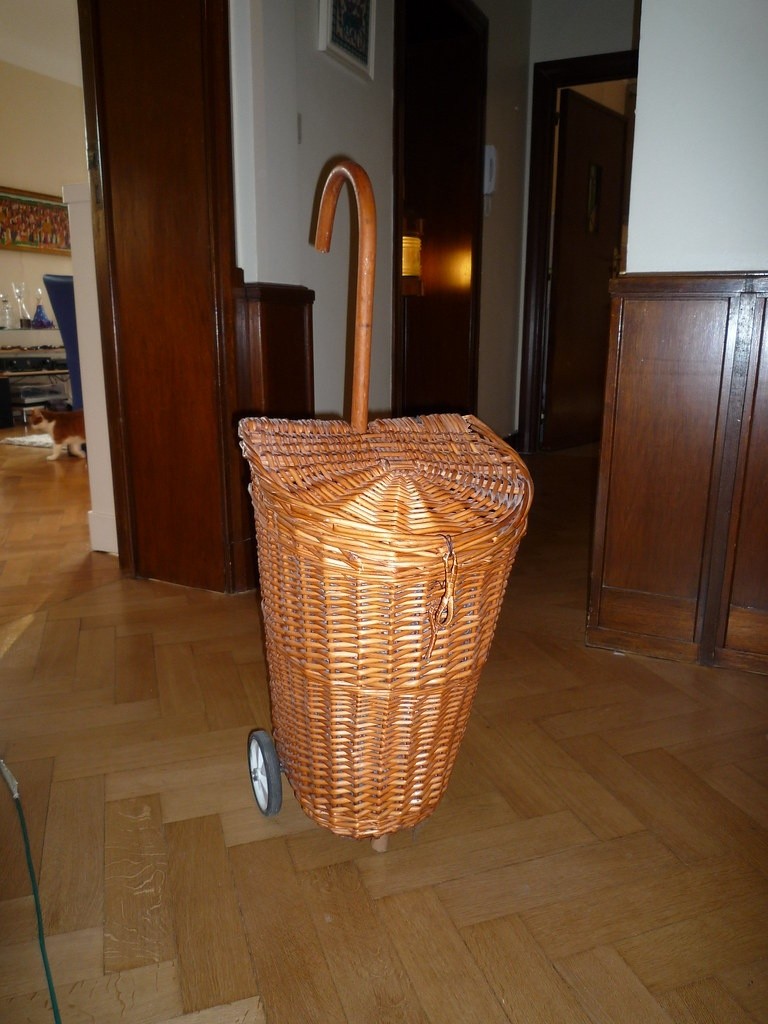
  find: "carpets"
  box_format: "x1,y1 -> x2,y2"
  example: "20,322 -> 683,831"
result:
0,433 -> 68,450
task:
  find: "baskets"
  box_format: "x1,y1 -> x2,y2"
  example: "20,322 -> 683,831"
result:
238,414 -> 533,839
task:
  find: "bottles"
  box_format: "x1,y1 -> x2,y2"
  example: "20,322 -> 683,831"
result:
0,300 -> 12,329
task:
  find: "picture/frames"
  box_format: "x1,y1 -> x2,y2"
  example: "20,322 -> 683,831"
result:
318,0 -> 378,84
0,186 -> 71,258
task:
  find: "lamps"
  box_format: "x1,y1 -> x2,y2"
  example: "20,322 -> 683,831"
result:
403,218 -> 425,294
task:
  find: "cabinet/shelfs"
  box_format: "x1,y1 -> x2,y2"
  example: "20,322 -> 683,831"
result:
0,329 -> 69,378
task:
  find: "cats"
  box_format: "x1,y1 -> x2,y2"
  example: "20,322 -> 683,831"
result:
28,408 -> 86,460
